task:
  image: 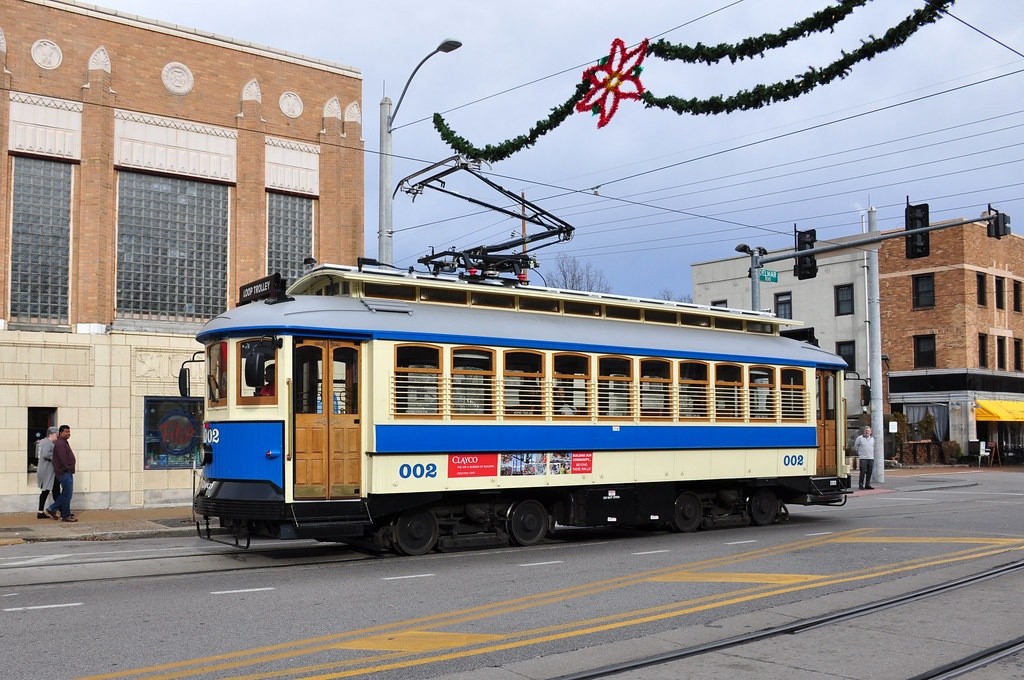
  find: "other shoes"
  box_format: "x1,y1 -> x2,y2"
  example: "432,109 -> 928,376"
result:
859,486 -> 864,490
46,508 -> 58,520
69,514 -> 74,517
37,512 -> 50,518
865,486 -> 874,489
61,517 -> 77,522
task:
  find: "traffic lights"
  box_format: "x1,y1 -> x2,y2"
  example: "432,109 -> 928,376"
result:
796,194 -> 1013,281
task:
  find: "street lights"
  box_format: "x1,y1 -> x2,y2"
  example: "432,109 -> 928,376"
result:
378,37 -> 462,272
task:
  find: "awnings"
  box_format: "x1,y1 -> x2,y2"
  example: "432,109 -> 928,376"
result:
975,399 -> 1024,421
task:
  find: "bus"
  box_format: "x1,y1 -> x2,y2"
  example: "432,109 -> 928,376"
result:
179,243 -> 856,560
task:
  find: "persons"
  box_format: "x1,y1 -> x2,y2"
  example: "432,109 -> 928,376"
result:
47,424 -> 78,522
553,385 -> 578,415
253,362 -> 275,396
520,381 -> 541,414
36,426 -> 75,519
854,425 -> 878,490
